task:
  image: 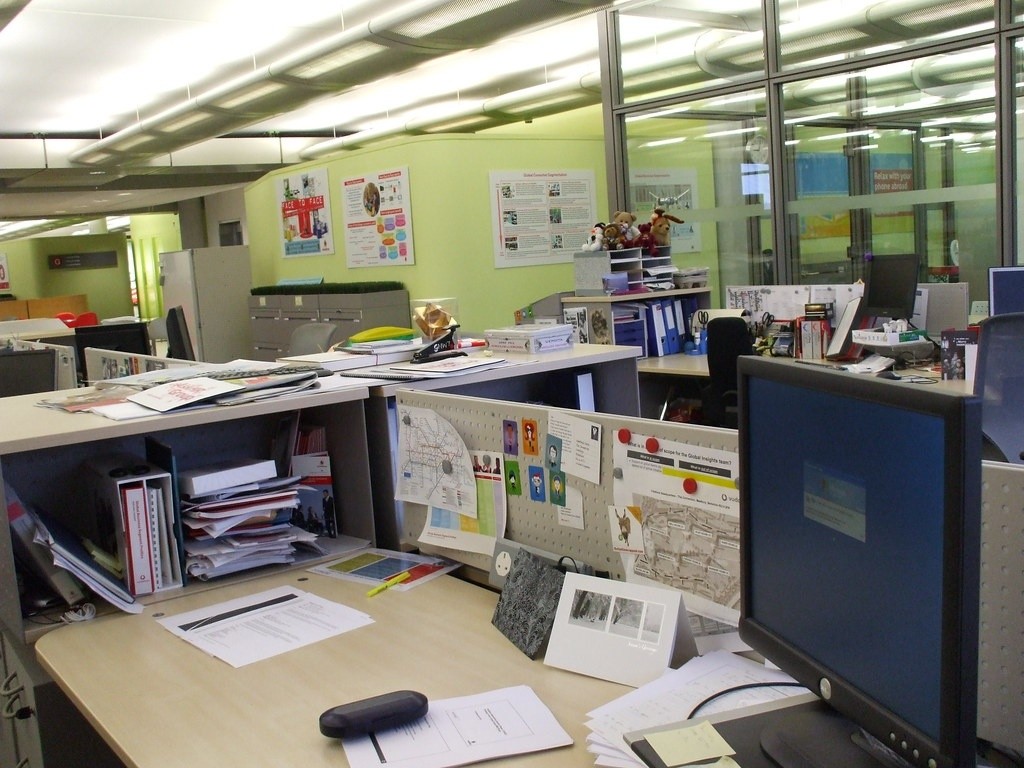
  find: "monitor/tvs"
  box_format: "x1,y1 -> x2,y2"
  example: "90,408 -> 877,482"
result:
862,253 -> 920,320
974,312 -> 1024,465
74,323 -> 153,388
989,266 -> 1024,317
734,356 -> 983,768
0,348 -> 59,398
165,306 -> 195,363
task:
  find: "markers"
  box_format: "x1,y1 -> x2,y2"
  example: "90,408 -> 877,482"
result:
458,341 -> 486,348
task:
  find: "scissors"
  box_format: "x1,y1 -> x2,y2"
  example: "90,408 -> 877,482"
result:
762,312 -> 775,330
697,311 -> 709,330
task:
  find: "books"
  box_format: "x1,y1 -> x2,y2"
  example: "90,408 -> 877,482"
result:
4,458 -> 325,615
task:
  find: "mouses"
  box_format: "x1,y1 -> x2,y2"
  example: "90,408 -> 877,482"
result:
874,371 -> 901,381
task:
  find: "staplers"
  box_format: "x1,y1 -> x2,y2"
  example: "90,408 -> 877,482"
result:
410,333 -> 468,365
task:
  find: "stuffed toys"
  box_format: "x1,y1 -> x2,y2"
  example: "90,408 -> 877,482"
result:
582,208 -> 684,257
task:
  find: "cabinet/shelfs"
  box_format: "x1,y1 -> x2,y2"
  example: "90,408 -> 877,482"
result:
0,246 -> 1024,768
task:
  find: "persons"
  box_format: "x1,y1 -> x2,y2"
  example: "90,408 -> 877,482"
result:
322,489 -> 336,538
307,505 -> 323,535
296,505 -> 305,529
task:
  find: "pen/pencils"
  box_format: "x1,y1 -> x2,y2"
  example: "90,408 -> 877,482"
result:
367,573 -> 411,598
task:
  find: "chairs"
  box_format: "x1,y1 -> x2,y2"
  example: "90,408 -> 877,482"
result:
702,317 -> 754,429
